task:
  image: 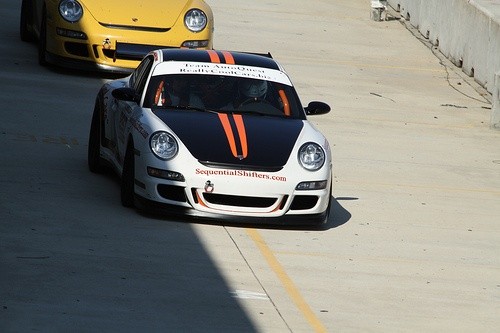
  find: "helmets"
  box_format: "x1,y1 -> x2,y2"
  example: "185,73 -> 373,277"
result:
239,78 -> 267,97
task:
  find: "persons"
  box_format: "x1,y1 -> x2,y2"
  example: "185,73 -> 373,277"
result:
223,80 -> 268,112
156,76 -> 205,111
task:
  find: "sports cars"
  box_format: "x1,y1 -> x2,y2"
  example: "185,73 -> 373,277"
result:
87,48 -> 332,229
20,0 -> 216,71
